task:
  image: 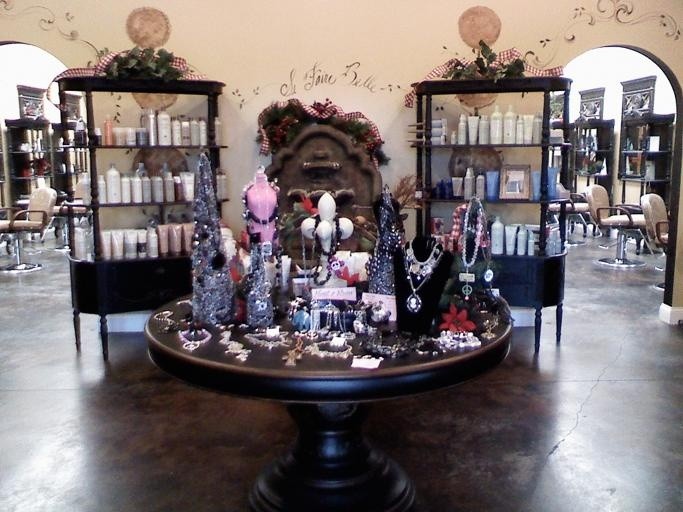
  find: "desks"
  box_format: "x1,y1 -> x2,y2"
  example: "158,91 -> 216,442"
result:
141,288 -> 511,512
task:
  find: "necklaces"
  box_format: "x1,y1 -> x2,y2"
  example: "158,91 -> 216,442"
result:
154,310 -> 482,368
313,216 -> 342,287
402,240 -> 445,312
241,182 -> 280,222
460,202 -> 483,302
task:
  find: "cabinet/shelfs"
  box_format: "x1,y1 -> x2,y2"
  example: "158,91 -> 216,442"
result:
55,77 -> 228,360
548,117 -> 614,177
617,113 -> 674,184
410,76 -> 572,350
5,116 -> 77,199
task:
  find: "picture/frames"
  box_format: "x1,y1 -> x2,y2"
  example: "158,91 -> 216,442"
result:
499,165 -> 531,203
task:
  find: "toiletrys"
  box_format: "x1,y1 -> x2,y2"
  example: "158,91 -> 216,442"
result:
436,166 -> 558,200
76,109 -> 221,146
452,104 -> 543,146
100,222 -> 194,261
492,216 -> 563,256
79,160 -> 228,203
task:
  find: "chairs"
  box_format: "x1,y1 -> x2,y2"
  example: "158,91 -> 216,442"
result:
547,183 -> 589,238
51,178 -> 91,217
640,193 -> 668,245
0,188 -> 57,273
586,183 -> 647,268
